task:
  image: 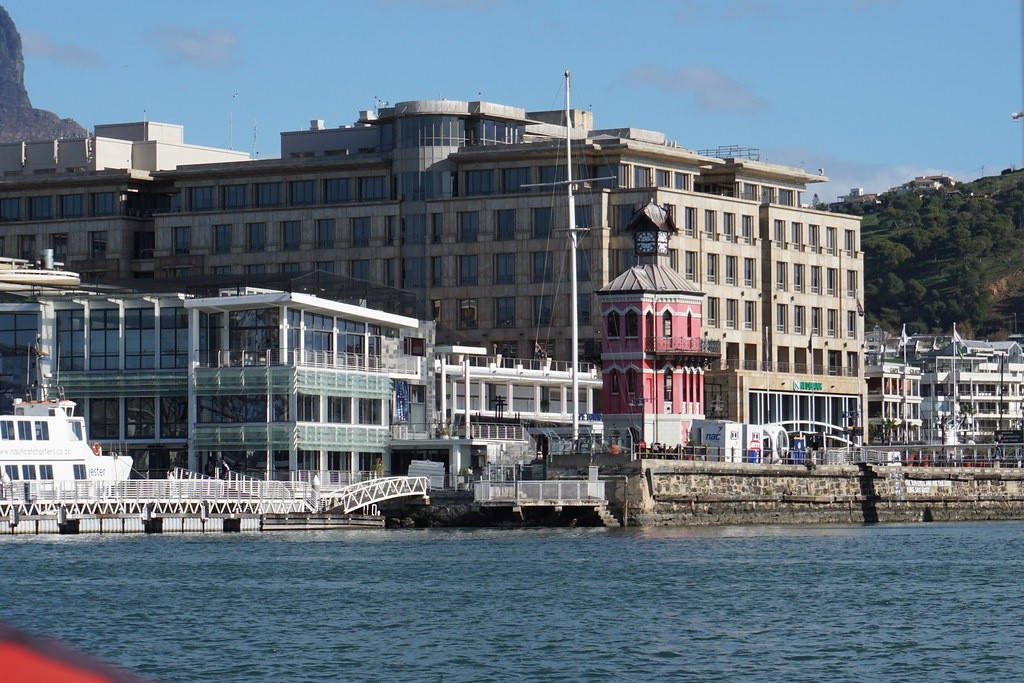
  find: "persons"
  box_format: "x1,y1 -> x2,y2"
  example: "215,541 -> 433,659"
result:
536,350 -> 546,369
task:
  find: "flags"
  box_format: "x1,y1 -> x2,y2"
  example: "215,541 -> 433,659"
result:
535,343 -> 544,357
900,329 -> 908,346
955,330 -> 964,349
857,298 -> 865,317
807,338 -> 812,354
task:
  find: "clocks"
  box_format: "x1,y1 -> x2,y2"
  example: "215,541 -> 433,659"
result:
634,231 -> 668,256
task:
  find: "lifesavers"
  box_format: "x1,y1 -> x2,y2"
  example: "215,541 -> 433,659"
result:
91,441 -> 101,456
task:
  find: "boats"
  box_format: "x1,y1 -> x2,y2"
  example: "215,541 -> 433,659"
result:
0,333 -> 134,506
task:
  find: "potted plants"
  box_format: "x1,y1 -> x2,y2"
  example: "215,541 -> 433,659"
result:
440,428 -> 450,439
963,448 -> 973,467
973,449 -> 986,466
685,441 -> 696,460
903,449 -> 915,466
917,447 -> 930,467
701,442 -> 711,460
451,431 -> 459,440
